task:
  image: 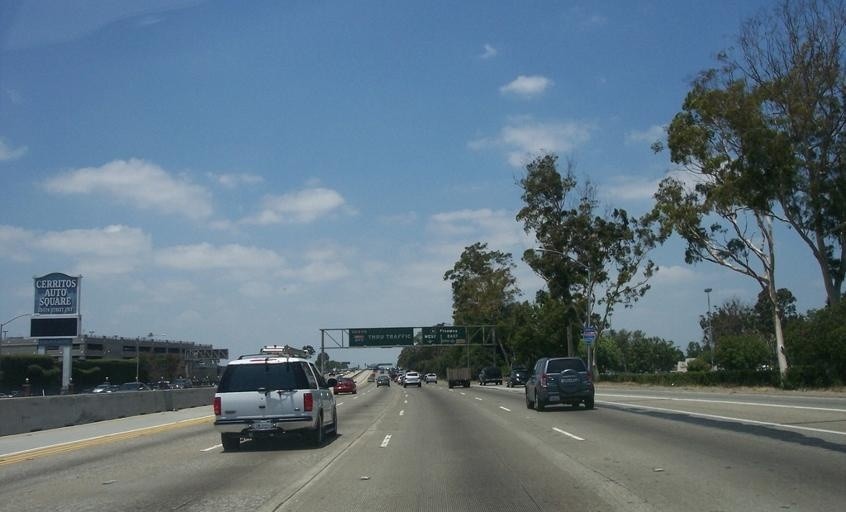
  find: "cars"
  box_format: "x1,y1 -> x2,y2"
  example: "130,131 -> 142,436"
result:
0,390 -> 21,399
327,366 -> 435,395
88,376 -> 219,394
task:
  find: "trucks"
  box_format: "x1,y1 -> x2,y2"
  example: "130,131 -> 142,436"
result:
446,367 -> 471,390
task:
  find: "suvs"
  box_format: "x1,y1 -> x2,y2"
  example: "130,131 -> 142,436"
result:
477,367 -> 503,386
522,356 -> 596,413
211,343 -> 338,454
504,368 -> 530,388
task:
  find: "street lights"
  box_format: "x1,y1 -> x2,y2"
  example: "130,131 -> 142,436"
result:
534,245 -> 592,379
0,312 -> 41,338
702,286 -> 715,374
135,333 -> 167,387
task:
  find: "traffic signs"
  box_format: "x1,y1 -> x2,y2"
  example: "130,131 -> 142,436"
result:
439,326 -> 466,345
420,327 -> 441,345
347,326 -> 414,348
